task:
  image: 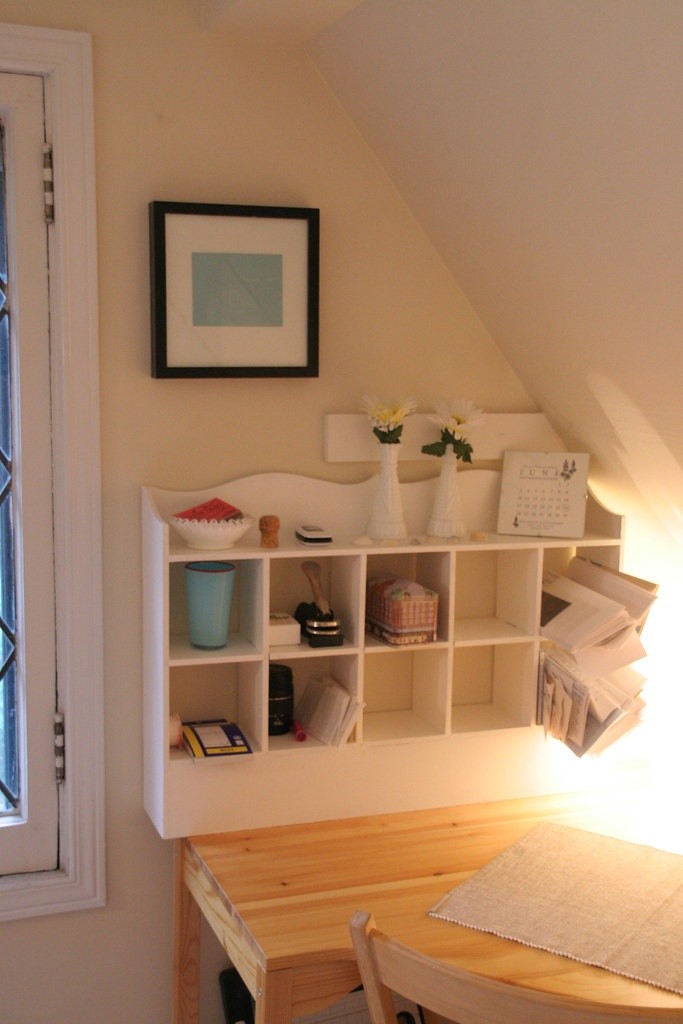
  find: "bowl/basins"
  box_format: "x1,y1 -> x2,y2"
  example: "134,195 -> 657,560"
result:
172,516 -> 253,550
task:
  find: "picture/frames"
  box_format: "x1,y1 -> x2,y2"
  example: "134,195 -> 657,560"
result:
149,200 -> 319,378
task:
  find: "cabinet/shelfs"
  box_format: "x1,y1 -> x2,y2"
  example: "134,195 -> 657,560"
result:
138,469 -> 626,841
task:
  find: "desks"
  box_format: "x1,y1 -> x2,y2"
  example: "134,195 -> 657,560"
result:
176,792 -> 683,1024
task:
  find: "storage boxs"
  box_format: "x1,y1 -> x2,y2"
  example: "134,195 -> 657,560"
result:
366,584 -> 437,645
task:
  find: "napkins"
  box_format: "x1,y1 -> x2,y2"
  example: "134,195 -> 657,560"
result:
428,819 -> 683,995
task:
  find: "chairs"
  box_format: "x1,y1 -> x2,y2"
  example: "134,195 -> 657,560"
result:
351,909 -> 683,1024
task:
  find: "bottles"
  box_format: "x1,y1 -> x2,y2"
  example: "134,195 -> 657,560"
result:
187,563 -> 235,650
268,664 -> 295,735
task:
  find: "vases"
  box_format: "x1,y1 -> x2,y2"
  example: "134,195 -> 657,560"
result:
426,447 -> 467,538
367,442 -> 408,540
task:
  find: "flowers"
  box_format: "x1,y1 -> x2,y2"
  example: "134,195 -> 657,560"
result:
362,394 -> 418,445
420,401 -> 484,462
